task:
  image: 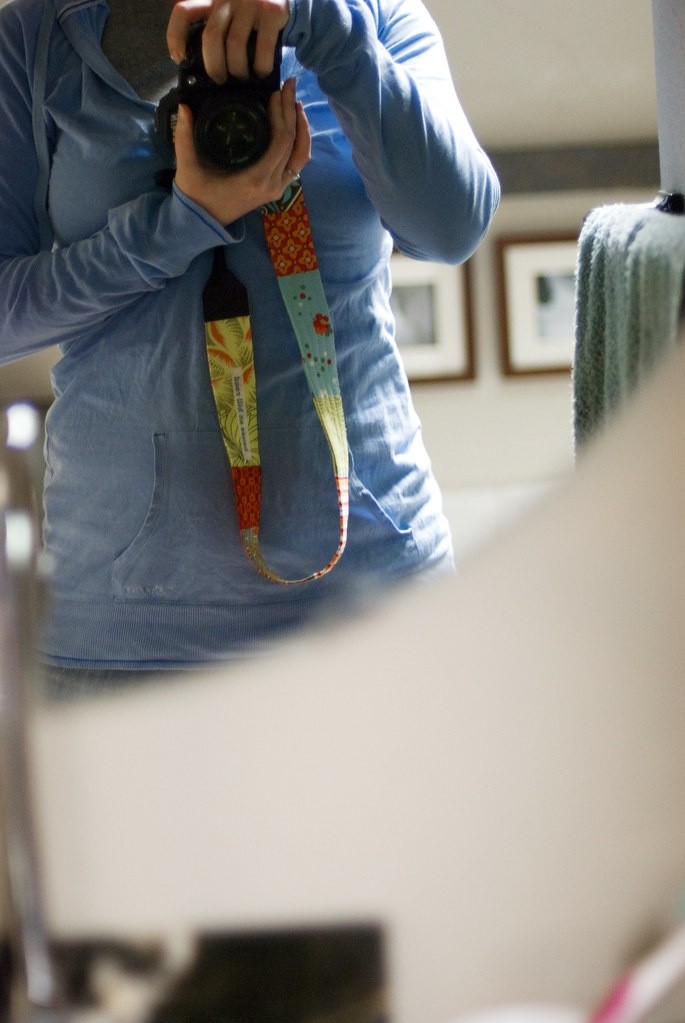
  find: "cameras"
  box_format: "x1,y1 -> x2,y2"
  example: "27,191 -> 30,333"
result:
154,16 -> 284,175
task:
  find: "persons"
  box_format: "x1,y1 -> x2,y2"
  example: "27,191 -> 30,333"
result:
0,0 -> 500,1023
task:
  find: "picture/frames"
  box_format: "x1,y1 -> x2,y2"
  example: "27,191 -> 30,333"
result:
495,233 -> 582,377
390,246 -> 476,386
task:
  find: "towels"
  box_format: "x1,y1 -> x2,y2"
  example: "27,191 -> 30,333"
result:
568,197 -> 685,470
